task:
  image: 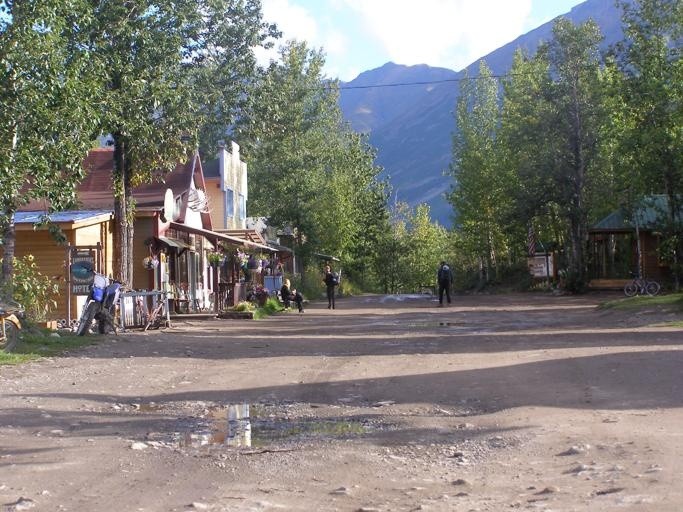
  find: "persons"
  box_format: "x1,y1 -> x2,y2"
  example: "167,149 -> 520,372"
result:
281,279 -> 305,313
323,266 -> 335,309
437,261 -> 453,306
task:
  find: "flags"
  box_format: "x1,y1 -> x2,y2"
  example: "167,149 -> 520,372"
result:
527,222 -> 536,257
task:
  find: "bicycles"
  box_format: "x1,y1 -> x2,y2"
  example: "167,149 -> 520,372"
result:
624,272 -> 660,297
143,291 -> 173,331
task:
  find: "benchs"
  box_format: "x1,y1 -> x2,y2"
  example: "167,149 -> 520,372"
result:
274,288 -> 297,311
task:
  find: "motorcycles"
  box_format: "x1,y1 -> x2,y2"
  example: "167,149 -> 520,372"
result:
77,266 -> 127,336
0,300 -> 25,353
